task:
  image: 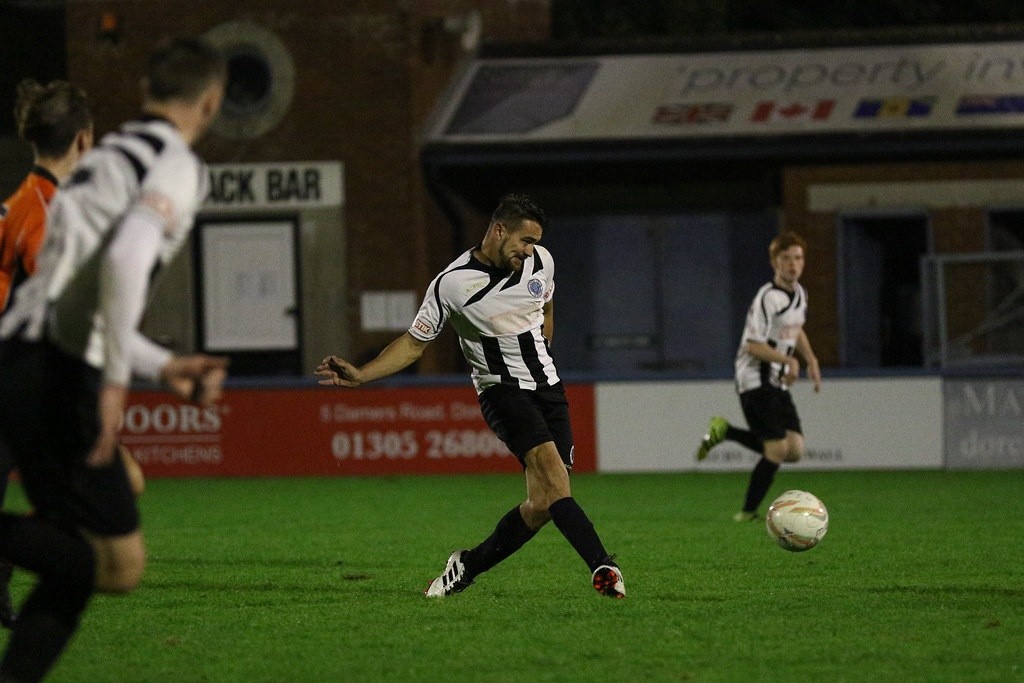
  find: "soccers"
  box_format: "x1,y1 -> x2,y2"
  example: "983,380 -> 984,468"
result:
766,491 -> 830,553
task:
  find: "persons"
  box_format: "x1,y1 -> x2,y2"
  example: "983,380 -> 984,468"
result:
0,33 -> 227,683
314,198 -> 626,599
0,78 -> 144,629
695,231 -> 821,523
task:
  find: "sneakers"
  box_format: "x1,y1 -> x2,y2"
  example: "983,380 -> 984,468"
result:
733,509 -> 760,523
591,553 -> 625,599
696,415 -> 728,461
425,548 -> 471,598
